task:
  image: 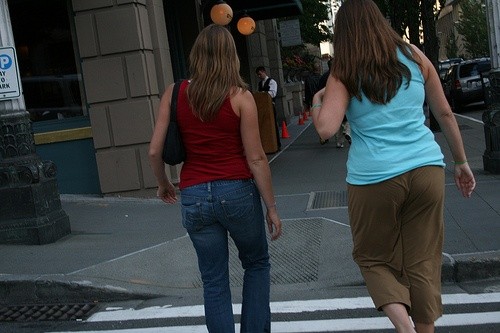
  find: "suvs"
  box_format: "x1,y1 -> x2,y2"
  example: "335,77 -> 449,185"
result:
442,58 -> 491,114
438,57 -> 463,80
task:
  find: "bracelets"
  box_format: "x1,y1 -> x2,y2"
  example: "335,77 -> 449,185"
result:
310,103 -> 322,112
452,158 -> 468,165
265,202 -> 277,208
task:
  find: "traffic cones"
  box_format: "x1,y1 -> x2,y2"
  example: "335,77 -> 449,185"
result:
308,105 -> 313,116
280,120 -> 289,138
303,109 -> 309,121
298,110 -> 305,125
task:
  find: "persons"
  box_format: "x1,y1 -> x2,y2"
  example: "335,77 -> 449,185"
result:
320,60 -> 352,149
147,24 -> 284,333
311,0 -> 477,333
255,64 -> 282,154
304,64 -> 330,145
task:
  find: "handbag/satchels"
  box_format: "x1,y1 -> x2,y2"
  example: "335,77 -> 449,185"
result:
161,79 -> 186,165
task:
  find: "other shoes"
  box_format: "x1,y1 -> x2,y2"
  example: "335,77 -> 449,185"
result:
336,142 -> 344,147
345,133 -> 352,143
